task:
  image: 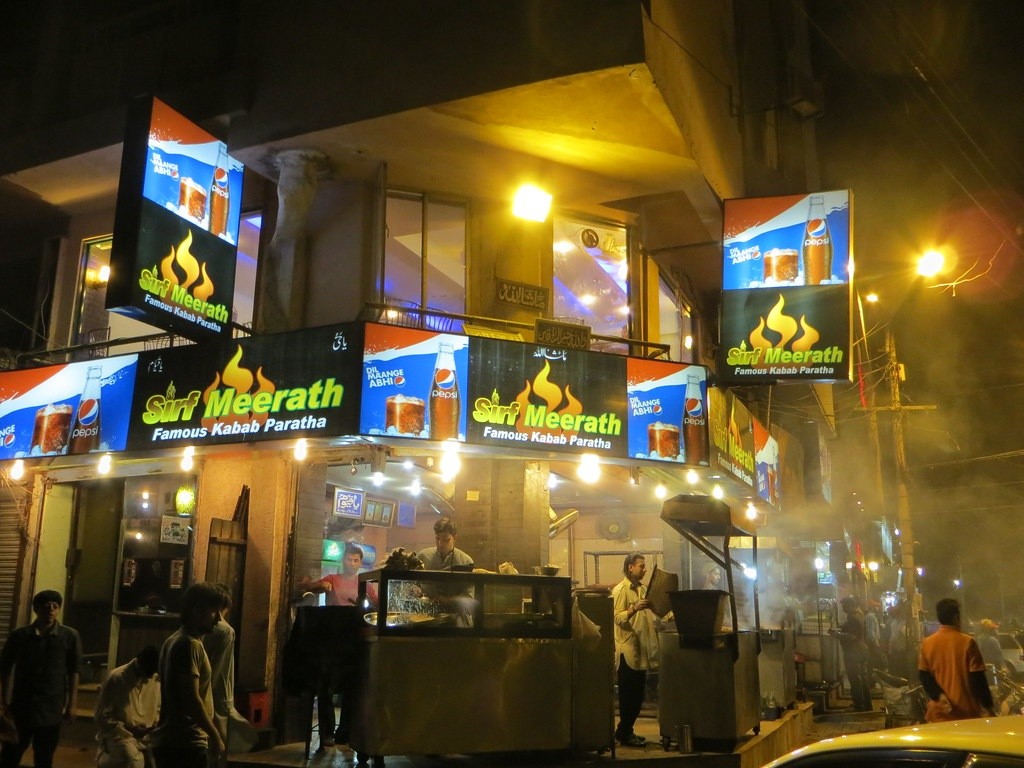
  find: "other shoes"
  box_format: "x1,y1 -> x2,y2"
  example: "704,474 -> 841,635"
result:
845,703 -> 873,712
615,728 -> 646,747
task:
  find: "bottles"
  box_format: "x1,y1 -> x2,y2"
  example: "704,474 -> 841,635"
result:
209,144 -> 230,236
430,341 -> 461,439
68,365 -> 102,454
802,195 -> 833,285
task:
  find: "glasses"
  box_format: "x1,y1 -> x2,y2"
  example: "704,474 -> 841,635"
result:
42,603 -> 59,609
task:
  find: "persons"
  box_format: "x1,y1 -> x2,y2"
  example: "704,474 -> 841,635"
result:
159,582 -> 229,768
917,598 -> 996,724
609,551 -> 673,748
298,545 -> 379,746
93,644 -> 161,768
0,590 -> 85,768
827,595 -> 1023,712
702,562 -> 721,590
410,517 -> 475,627
202,585 -> 258,767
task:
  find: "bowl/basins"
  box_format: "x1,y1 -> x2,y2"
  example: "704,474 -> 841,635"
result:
538,567 -> 559,576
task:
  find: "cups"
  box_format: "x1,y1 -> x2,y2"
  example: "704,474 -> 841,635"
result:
180,178 -> 207,222
387,397 -> 425,435
682,375 -> 708,464
763,250 -> 799,282
767,468 -> 779,506
32,405 -> 72,453
647,424 -> 680,460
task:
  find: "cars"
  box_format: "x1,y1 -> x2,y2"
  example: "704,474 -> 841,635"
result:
996,632 -> 1024,681
761,715 -> 1024,768
919,621 -> 943,637
